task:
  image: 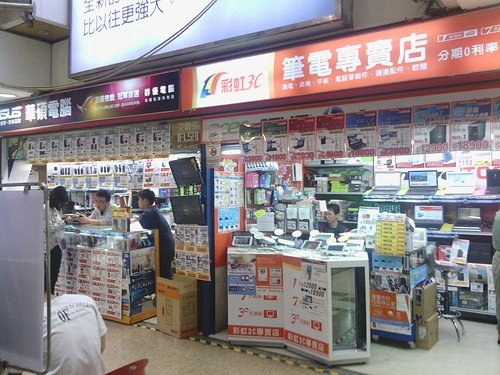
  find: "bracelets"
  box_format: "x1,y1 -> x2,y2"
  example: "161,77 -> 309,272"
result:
64,220 -> 68,224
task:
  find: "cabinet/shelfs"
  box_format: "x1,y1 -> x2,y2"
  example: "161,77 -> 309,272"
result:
305,158 -> 500,325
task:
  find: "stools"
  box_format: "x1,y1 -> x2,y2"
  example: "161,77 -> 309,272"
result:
427,242 -> 467,341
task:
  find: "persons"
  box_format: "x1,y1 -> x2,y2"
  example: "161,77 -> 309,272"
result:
73,189 -> 117,226
324,203 -> 349,239
492,209 -> 500,344
44,186 -> 72,294
129,189 -> 175,306
29,267 -> 108,375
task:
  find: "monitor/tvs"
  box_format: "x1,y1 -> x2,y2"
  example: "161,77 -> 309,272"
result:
168,156 -> 204,186
170,195 -> 205,225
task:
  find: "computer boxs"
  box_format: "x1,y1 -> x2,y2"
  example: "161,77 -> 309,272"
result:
430,125 -> 447,144
468,121 -> 486,142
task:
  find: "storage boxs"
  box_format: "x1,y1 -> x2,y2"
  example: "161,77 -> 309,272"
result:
155,274 -> 198,339
313,174 -> 495,350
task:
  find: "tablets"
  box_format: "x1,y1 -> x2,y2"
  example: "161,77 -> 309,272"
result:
233,236 -> 346,252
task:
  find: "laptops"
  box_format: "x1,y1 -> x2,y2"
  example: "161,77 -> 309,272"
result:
369,168 -> 500,230
243,134 -> 367,154
